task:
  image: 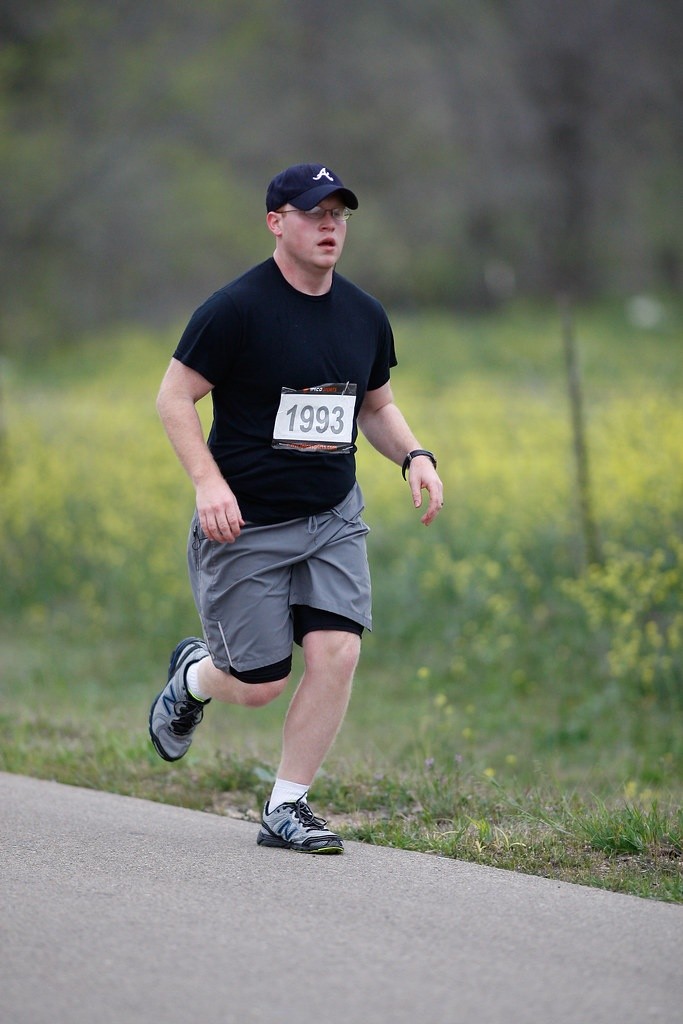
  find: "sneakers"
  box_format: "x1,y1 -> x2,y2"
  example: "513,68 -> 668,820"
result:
148,637 -> 215,761
257,795 -> 344,854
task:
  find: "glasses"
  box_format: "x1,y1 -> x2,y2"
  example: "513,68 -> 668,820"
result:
270,205 -> 354,221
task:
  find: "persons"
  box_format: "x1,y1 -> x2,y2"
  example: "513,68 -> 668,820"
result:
148,163 -> 443,854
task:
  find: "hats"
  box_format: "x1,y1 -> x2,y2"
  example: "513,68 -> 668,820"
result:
266,163 -> 359,219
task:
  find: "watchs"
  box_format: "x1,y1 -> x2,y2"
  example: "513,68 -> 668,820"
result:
401,449 -> 437,481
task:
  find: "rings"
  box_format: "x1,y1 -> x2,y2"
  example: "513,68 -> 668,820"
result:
440,503 -> 444,506
